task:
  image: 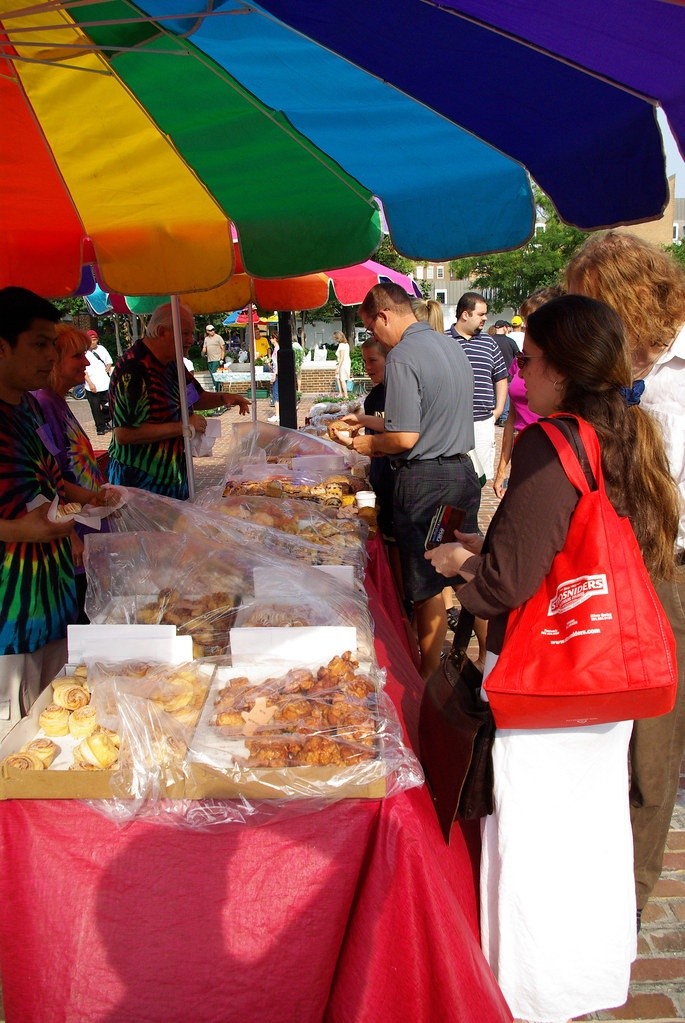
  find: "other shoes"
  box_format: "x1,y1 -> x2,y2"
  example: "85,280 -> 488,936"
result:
495,418 -> 507,426
269,400 -> 275,407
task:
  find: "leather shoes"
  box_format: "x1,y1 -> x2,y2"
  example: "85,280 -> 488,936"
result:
104,423 -> 111,430
97,430 -> 105,435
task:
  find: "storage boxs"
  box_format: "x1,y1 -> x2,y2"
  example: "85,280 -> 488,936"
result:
94,450 -> 110,482
247,388 -> 269,398
292,455 -> 345,471
0,519 -> 386,798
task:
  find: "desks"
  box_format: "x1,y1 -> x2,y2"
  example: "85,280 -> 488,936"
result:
0,527 -> 513,1023
212,372 -> 277,392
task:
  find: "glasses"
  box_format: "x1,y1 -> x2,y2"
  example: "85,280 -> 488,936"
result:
515,350 -> 548,369
365,308 -> 391,336
208,328 -> 215,332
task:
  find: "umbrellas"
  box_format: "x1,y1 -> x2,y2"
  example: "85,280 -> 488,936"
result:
83,259 -> 423,423
222,303 -> 279,360
0,0 -> 685,503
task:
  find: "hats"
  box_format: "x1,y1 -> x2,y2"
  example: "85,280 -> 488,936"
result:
87,330 -> 98,338
495,320 -> 506,328
206,325 -> 214,331
512,315 -> 522,327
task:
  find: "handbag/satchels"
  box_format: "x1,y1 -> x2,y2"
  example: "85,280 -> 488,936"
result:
314,345 -> 327,361
417,651 -> 495,845
482,413 -> 678,728
353,382 -> 364,395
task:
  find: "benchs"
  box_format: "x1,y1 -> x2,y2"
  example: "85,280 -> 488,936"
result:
352,377 -> 372,396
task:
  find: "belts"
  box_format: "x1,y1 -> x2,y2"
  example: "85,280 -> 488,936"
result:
411,454 -> 466,464
674,551 -> 685,566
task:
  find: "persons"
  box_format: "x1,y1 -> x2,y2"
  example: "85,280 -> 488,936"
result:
424,296 -> 679,1022
0,234 -> 685,1023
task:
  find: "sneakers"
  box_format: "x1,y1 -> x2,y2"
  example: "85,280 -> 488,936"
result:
267,415 -> 279,422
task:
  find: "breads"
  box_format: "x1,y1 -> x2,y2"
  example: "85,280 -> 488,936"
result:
56,451 -> 372,660
5,650 -> 377,771
303,402 -> 364,439
